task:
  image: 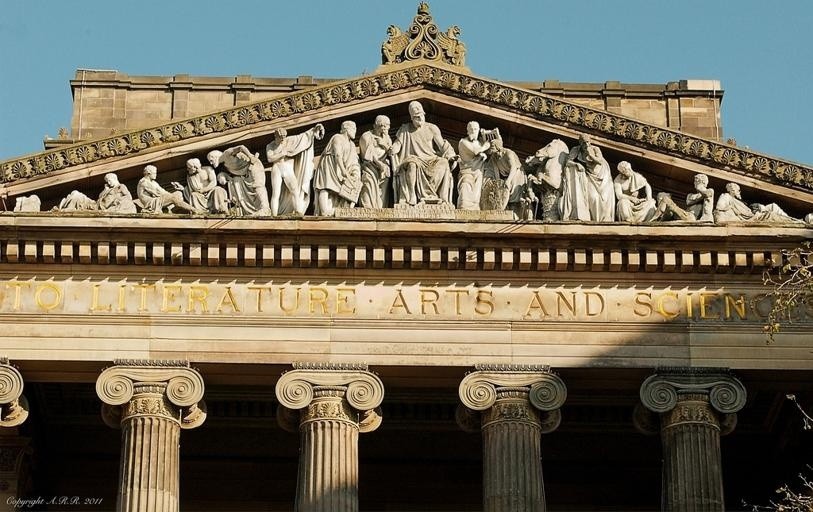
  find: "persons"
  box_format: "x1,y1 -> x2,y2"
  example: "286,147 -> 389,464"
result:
57,100 -> 804,222
